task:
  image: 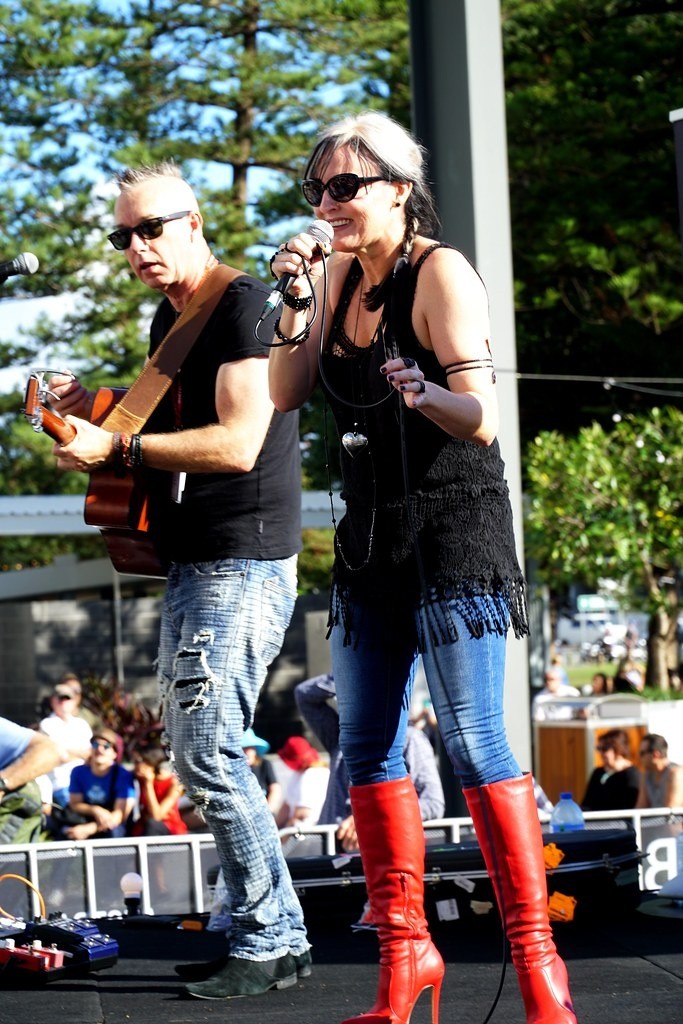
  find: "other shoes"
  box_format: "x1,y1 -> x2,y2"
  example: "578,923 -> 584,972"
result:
290,951 -> 312,979
185,949 -> 298,1000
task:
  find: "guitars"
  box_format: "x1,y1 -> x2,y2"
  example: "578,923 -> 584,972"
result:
22,377 -> 175,578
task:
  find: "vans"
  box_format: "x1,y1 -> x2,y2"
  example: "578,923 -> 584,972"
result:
554,611 -> 612,647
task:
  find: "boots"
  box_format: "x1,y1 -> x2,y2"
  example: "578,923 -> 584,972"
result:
342,774 -> 446,1024
466,772 -> 577,1024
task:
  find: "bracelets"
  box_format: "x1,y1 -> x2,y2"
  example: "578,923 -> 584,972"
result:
273,317 -> 310,345
112,431 -> 143,467
282,292 -> 313,310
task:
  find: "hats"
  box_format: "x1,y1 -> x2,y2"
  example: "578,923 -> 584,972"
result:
89,728 -> 124,765
240,727 -> 270,756
278,735 -> 321,771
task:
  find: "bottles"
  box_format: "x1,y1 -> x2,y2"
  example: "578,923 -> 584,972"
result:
549,792 -> 585,832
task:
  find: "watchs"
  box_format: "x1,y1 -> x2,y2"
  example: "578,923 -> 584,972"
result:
0,777 -> 11,793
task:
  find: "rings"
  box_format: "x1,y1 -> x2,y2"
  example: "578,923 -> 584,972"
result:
403,358 -> 415,369
285,242 -> 292,252
416,380 -> 426,393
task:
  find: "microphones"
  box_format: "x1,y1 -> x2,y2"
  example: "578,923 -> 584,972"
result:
0,252 -> 39,278
260,220 -> 334,320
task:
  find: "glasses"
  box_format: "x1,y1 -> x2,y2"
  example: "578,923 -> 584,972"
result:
300,173 -> 386,208
105,210 -> 193,251
90,741 -> 111,750
59,696 -> 70,702
640,749 -> 652,756
595,743 -> 613,752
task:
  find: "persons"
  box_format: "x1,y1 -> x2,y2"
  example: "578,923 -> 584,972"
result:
293,672 -> 445,854
277,737 -> 330,826
551,657 -> 569,685
580,729 -> 642,812
242,728 -> 290,825
268,112 -> 578,1024
636,733 -> 683,809
0,716 -> 64,803
531,669 -> 581,722
39,673 -> 94,808
47,728 -> 137,906
134,742 -> 188,892
49,162 -> 313,1000
593,663 -> 633,693
